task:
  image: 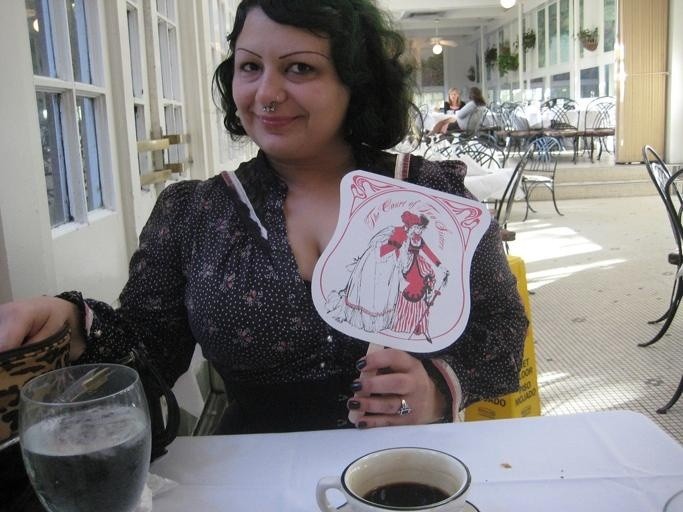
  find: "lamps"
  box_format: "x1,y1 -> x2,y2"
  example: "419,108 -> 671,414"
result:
500,0 -> 516,9
433,43 -> 442,55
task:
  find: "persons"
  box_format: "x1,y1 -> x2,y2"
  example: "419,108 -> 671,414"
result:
440,87 -> 486,138
0,1 -> 529,429
443,87 -> 465,114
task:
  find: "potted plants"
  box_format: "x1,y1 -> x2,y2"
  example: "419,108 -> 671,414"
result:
577,28 -> 599,51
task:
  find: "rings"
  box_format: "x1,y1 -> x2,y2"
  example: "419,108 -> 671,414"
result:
400,397 -> 412,416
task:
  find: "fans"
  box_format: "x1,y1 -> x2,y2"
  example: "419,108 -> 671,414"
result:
420,36 -> 456,47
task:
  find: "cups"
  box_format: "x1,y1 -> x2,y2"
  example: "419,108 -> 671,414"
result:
315,447 -> 473,512
18,363 -> 152,512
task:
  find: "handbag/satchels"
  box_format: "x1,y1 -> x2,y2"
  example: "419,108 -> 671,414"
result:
1,320 -> 180,512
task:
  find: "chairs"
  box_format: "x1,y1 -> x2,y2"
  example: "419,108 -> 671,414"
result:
638,145 -> 683,348
656,168 -> 682,413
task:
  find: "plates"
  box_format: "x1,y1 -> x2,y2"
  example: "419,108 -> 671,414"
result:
336,500 -> 479,511
663,489 -> 682,511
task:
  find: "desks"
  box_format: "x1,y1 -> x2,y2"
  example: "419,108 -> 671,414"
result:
143,411 -> 683,511
